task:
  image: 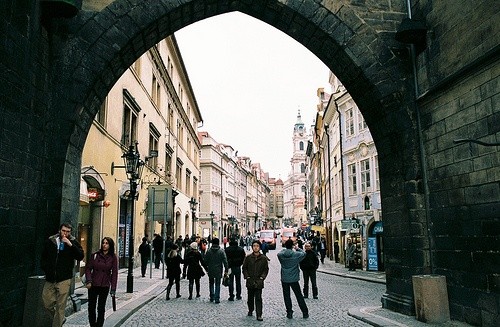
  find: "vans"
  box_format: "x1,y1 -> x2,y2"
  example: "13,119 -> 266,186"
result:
257,230 -> 277,250
280,228 -> 297,247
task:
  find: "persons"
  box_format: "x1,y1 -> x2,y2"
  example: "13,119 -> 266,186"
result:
165,244 -> 184,300
182,242 -> 208,300
138,237 -> 150,277
226,240 -> 246,301
176,234 -> 211,258
300,244 -> 318,299
41,222 -> 84,327
152,234 -> 163,269
243,240 -> 269,321
205,238 -> 229,304
319,238 -> 326,264
277,239 -> 309,319
165,235 -> 175,270
368,240 -> 376,253
85,237 -> 118,327
346,239 -> 355,271
288,229 -> 321,255
223,233 -> 244,248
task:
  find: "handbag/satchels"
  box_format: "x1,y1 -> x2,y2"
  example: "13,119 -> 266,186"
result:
81,270 -> 93,286
223,274 -> 230,286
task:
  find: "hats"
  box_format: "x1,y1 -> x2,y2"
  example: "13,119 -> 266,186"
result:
172,243 -> 178,250
190,242 -> 198,249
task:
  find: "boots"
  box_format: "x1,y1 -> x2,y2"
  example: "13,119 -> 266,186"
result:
188,285 -> 193,300
176,284 -> 181,298
196,284 -> 200,297
166,285 -> 171,300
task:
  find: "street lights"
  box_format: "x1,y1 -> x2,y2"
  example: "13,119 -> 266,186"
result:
188,196 -> 199,236
121,139 -> 144,294
210,211 -> 214,240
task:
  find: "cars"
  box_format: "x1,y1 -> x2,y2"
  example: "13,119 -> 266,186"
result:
255,227 -> 298,238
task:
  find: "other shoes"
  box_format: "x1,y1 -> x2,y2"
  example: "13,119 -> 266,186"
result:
210,297 -> 214,301
303,295 -> 308,298
303,312 -> 309,318
287,314 -> 292,318
313,295 -> 318,299
257,316 -> 263,320
215,299 -> 220,303
248,310 -> 253,316
228,297 -> 233,300
236,296 -> 242,299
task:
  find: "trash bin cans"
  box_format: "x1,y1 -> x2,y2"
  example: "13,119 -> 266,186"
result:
412,274 -> 449,324
22,276 -> 54,327
